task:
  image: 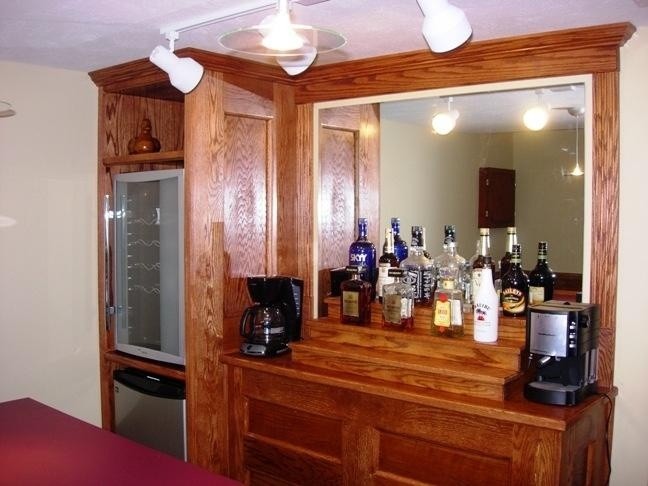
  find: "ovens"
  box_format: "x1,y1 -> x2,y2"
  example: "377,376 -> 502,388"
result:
107,365 -> 189,465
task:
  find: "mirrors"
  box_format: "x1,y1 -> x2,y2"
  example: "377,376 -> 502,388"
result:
309,70 -> 598,333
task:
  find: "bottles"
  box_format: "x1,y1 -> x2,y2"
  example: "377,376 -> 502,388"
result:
499,242 -> 528,317
432,224 -> 466,303
348,216 -> 431,303
470,258 -> 497,343
526,238 -> 556,311
497,222 -> 521,270
432,274 -> 464,338
378,265 -> 415,330
469,228 -> 498,272
127,118 -> 161,154
338,264 -> 370,326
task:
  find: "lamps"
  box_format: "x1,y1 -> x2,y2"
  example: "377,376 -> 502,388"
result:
430,96 -> 462,136
521,86 -> 553,134
218,2 -> 352,80
417,0 -> 474,57
568,102 -> 585,178
149,24 -> 205,95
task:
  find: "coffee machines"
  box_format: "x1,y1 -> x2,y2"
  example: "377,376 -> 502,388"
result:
514,300 -> 603,406
239,274 -> 305,358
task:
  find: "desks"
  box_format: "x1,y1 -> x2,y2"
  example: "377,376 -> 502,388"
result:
214,347 -> 621,486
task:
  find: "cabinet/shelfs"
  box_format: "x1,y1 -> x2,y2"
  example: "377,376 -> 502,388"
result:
85,46 -> 280,480
478,166 -> 517,229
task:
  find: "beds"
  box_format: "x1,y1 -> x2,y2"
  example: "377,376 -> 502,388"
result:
1,397 -> 246,486
